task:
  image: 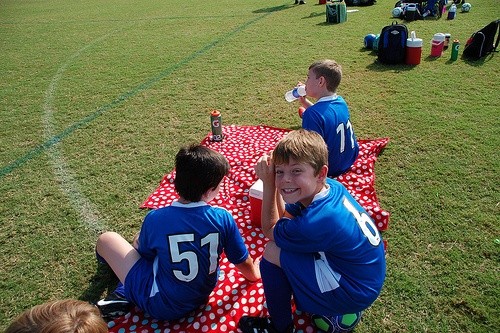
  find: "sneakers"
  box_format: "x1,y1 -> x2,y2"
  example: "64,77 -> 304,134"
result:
93,292 -> 132,318
240,316 -> 297,333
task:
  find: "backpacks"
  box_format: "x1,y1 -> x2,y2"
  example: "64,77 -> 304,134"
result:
378,21 -> 408,64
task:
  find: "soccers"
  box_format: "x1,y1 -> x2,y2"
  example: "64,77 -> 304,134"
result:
314,311 -> 361,333
392,7 -> 402,18
461,2 -> 471,12
363,34 -> 381,51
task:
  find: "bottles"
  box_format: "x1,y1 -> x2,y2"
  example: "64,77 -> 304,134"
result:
284,85 -> 306,102
451,39 -> 459,61
211,110 -> 223,142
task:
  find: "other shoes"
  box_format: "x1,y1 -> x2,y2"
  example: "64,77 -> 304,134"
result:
300,1 -> 306,4
294,2 -> 298,4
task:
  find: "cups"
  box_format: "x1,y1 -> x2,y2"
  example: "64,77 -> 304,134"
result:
443,33 -> 451,50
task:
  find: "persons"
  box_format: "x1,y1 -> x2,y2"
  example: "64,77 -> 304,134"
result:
296,59 -> 359,177
239,129 -> 386,333
4,300 -> 109,333
95,144 -> 261,322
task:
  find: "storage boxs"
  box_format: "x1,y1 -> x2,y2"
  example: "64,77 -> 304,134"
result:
250,178 -> 263,227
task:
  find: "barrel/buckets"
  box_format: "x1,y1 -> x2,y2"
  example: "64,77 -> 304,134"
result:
447,12 -> 455,19
431,32 -> 445,57
406,30 -> 423,65
450,4 -> 457,18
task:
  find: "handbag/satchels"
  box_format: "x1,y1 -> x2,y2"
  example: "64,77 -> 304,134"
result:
326,3 -> 347,23
423,0 -> 439,16
353,0 -> 376,6
464,19 -> 500,58
453,0 -> 465,5
